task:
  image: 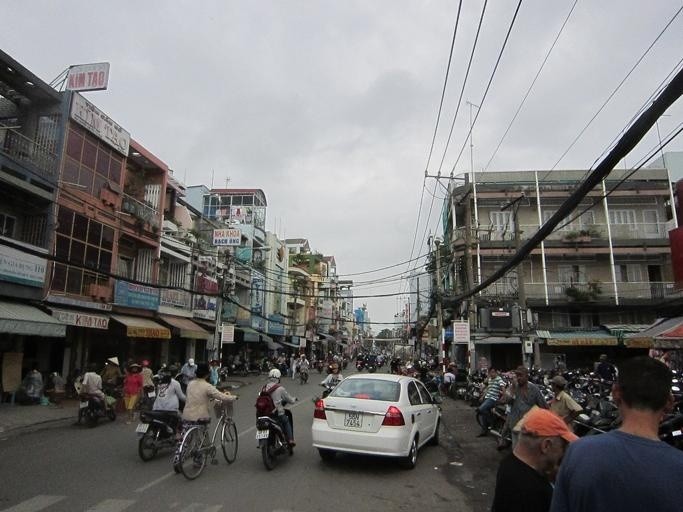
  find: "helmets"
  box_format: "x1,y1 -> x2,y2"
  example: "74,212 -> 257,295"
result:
267,368 -> 282,380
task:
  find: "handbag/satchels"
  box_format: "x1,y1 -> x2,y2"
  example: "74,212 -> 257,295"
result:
114,395 -> 126,413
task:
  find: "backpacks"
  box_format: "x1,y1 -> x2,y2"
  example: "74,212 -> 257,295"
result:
255,383 -> 282,416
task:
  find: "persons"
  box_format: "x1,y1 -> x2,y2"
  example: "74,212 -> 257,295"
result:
490,407 -> 579,511
15,350 -> 682,474
548,354 -> 682,511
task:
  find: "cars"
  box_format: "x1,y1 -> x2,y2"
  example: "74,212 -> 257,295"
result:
311,372 -> 442,469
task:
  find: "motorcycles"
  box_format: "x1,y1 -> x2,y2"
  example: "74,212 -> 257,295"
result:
354,352 -> 385,373
415,360 -> 682,451
229,355 -> 348,382
254,398 -> 299,471
210,358 -> 228,382
134,404 -> 184,461
317,382 -> 335,398
74,363 -> 181,428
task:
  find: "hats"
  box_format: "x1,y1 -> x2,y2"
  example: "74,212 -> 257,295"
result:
195,363 -> 211,376
549,375 -> 566,387
127,362 -> 143,373
514,365 -> 528,374
521,408 -> 581,444
107,356 -> 120,367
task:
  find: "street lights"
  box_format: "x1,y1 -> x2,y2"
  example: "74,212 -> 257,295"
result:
347,333 -> 362,337
426,234 -> 443,366
213,246 -> 271,363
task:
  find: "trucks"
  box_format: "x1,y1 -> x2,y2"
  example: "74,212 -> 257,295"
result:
389,344 -> 414,366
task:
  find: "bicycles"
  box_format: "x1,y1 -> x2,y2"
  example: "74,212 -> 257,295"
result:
170,397 -> 239,481
